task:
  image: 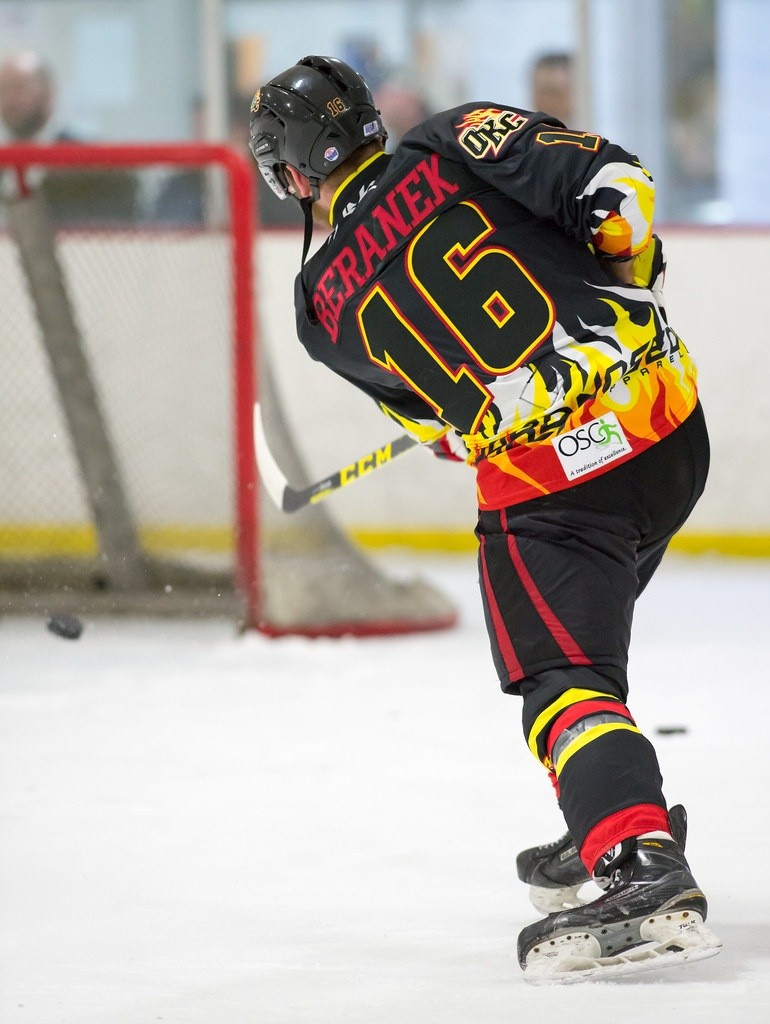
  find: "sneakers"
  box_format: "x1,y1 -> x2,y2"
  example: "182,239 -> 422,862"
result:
516,804 -> 688,915
517,835 -> 722,985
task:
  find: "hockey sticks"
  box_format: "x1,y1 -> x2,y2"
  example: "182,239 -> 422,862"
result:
252,401 -> 417,515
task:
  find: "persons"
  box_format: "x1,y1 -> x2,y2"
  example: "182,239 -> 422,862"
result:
249,53 -> 727,982
1,43 -> 573,230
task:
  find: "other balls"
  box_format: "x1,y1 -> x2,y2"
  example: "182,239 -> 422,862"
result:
46,613 -> 84,640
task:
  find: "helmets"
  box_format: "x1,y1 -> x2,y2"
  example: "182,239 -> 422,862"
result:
249,55 -> 389,200
